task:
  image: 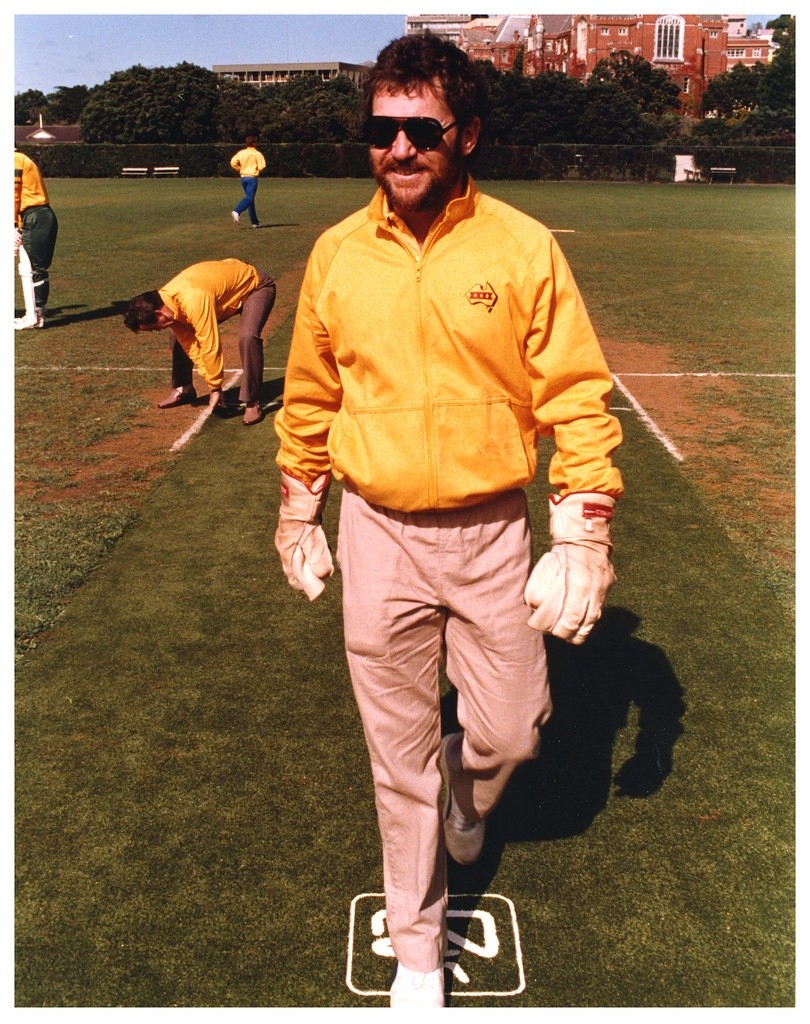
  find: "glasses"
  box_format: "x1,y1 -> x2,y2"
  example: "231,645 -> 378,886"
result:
363,115 -> 462,150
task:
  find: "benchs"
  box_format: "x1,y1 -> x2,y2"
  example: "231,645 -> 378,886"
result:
153,167 -> 179,177
708,167 -> 737,185
121,167 -> 148,177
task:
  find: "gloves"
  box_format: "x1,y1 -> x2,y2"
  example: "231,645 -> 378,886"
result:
524,490 -> 619,646
15,220 -> 23,257
273,469 -> 336,603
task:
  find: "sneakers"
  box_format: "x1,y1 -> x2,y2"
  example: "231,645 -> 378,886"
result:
231,210 -> 241,227
15,307 -> 45,329
243,402 -> 264,426
389,961 -> 445,1008
440,732 -> 488,867
156,388 -> 197,409
252,223 -> 263,228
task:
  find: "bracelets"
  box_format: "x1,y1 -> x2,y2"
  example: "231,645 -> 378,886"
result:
211,388 -> 221,392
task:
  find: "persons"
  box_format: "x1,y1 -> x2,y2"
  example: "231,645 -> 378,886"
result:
230,136 -> 266,227
272,32 -> 623,1008
124,257 -> 277,425
14,152 -> 58,330
